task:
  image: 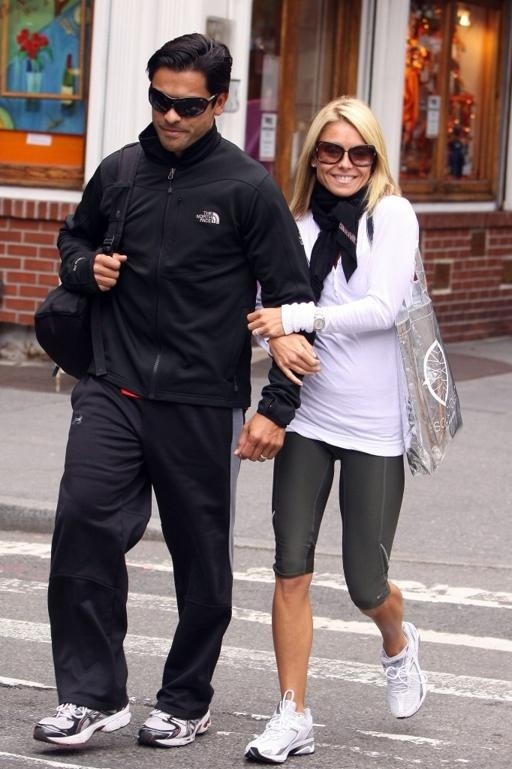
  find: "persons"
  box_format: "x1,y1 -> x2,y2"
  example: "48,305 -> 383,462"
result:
26,31 -> 314,752
247,93 -> 462,762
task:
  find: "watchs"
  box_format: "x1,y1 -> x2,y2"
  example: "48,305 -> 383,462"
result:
314,306 -> 326,332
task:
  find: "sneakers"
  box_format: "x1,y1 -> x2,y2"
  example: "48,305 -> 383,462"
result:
379,621 -> 427,720
33,701 -> 132,746
136,707 -> 212,749
245,700 -> 316,762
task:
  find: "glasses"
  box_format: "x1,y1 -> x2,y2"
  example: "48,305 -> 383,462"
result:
148,82 -> 217,118
315,139 -> 378,167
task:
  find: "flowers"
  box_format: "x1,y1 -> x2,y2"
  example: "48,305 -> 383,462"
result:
16,29 -> 54,71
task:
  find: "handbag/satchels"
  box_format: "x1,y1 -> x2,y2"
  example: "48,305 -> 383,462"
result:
394,281 -> 463,476
33,281 -> 92,381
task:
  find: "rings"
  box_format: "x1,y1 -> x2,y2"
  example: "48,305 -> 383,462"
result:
260,454 -> 268,460
253,328 -> 260,337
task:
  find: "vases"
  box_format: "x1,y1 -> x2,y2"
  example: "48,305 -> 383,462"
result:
27,73 -> 40,93
